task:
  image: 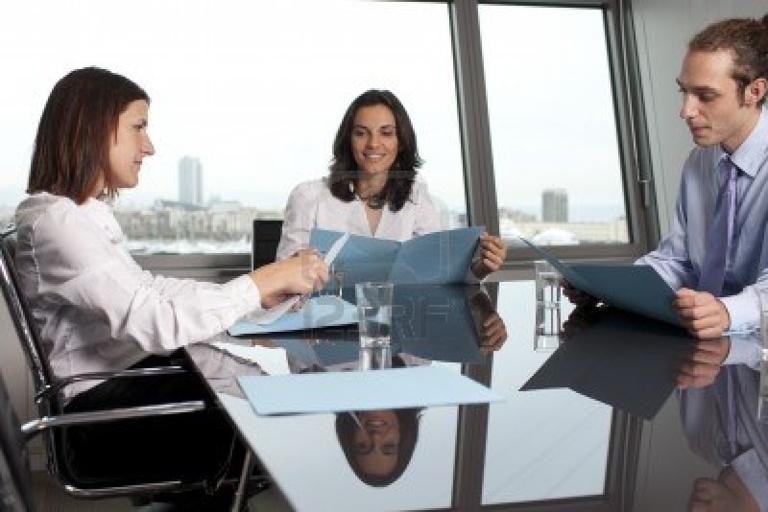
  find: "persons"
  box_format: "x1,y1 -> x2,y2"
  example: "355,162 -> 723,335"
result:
285,285 -> 507,489
18,65 -> 334,511
275,89 -> 509,283
668,329 -> 768,509
564,12 -> 767,341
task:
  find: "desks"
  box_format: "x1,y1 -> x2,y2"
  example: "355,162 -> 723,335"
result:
182,276 -> 766,512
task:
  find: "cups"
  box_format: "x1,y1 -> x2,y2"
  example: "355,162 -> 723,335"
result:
317,253 -> 343,299
756,364 -> 767,421
358,347 -> 393,372
533,306 -> 562,354
356,282 -> 393,350
760,304 -> 768,363
532,259 -> 564,306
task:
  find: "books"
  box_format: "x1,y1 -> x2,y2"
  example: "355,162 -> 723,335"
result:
515,233 -> 693,326
236,357 -> 509,416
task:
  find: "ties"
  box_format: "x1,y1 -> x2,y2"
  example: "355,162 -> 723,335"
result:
711,363 -> 738,465
698,163 -> 740,296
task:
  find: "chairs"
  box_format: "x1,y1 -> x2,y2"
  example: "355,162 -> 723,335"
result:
250,216 -> 285,274
0,254 -> 205,512
4,248 -> 186,496
